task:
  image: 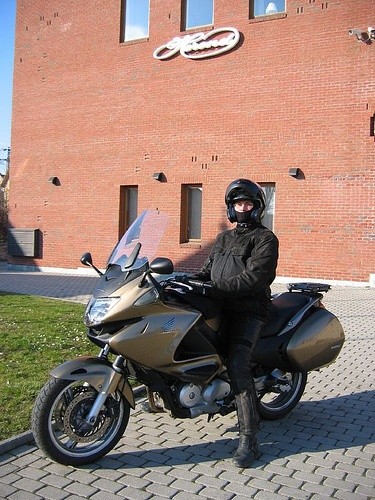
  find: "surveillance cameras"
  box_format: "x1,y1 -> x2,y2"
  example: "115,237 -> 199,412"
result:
349,29 -> 362,35
368,27 -> 375,34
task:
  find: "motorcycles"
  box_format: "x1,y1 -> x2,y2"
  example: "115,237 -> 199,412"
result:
31,209 -> 345,466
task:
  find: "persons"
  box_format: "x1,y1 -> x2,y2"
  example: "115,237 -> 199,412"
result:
140,179 -> 279,469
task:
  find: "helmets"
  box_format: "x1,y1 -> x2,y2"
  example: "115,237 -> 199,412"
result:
225,179 -> 267,222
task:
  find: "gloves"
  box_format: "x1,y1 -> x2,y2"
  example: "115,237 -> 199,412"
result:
175,274 -> 189,286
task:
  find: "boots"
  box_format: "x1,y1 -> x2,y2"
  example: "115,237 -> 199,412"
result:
233,383 -> 256,468
